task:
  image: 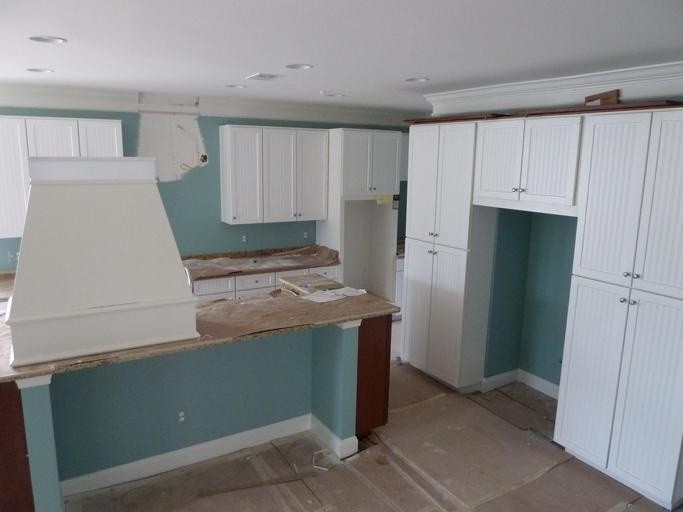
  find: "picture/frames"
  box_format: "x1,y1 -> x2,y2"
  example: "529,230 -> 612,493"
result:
0,274 -> 404,512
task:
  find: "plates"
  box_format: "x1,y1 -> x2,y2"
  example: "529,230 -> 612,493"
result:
192,272 -> 276,304
315,128 -> 407,323
356,314 -> 392,436
276,263 -> 342,291
219,123 -> 331,226
0,115 -> 125,239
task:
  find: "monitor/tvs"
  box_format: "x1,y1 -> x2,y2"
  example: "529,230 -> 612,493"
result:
278,273 -> 345,296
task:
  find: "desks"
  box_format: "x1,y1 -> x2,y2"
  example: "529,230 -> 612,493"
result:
0,274 -> 404,512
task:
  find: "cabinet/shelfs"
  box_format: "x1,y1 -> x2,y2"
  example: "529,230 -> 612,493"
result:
356,314 -> 392,436
315,128 -> 407,323
276,263 -> 342,291
473,115 -> 585,217
403,119 -> 497,393
219,123 -> 331,226
0,115 -> 125,239
192,272 -> 276,304
554,108 -> 682,510
